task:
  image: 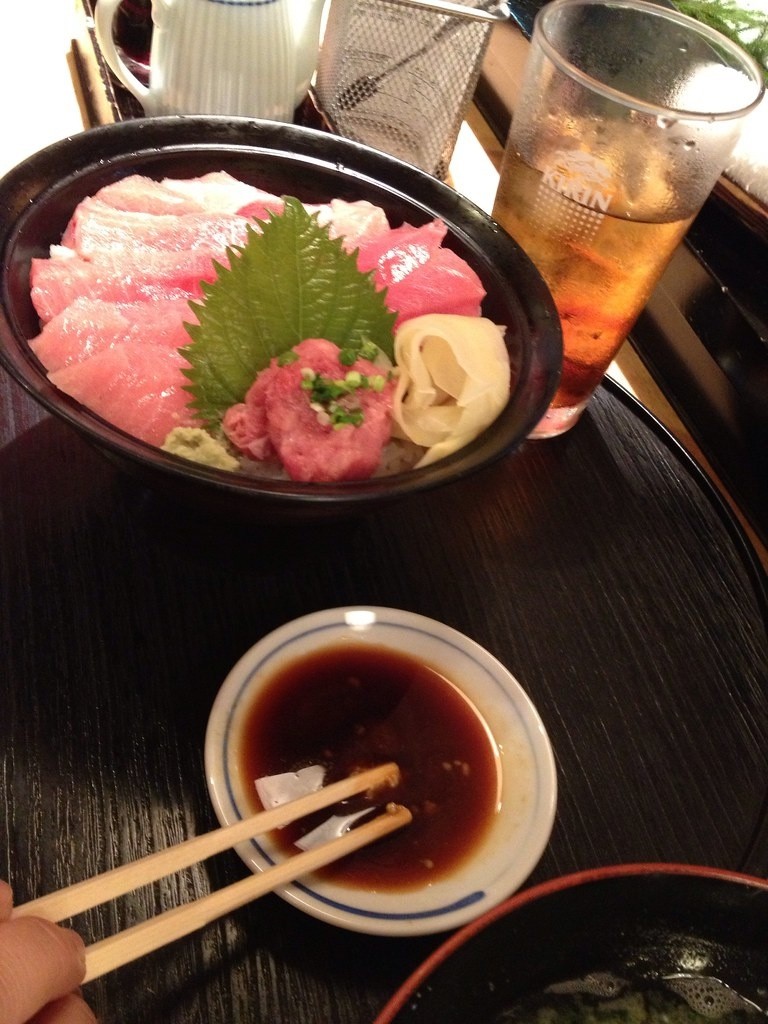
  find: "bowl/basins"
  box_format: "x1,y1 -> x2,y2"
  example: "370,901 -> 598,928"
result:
207,605 -> 559,937
1,115 -> 565,504
376,860 -> 767,1024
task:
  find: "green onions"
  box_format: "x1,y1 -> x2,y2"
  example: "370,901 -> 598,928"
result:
276,340 -> 394,433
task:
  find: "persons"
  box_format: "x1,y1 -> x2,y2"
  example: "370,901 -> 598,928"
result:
0,881 -> 98,1024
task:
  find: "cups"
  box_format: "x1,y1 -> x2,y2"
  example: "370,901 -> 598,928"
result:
492,1 -> 767,438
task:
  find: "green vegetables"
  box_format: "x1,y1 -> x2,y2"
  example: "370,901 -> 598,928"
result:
177,194 -> 401,438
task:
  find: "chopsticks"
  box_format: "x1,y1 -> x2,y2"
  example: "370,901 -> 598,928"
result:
9,761 -> 413,985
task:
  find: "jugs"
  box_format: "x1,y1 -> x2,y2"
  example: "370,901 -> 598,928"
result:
94,0 -> 325,125
